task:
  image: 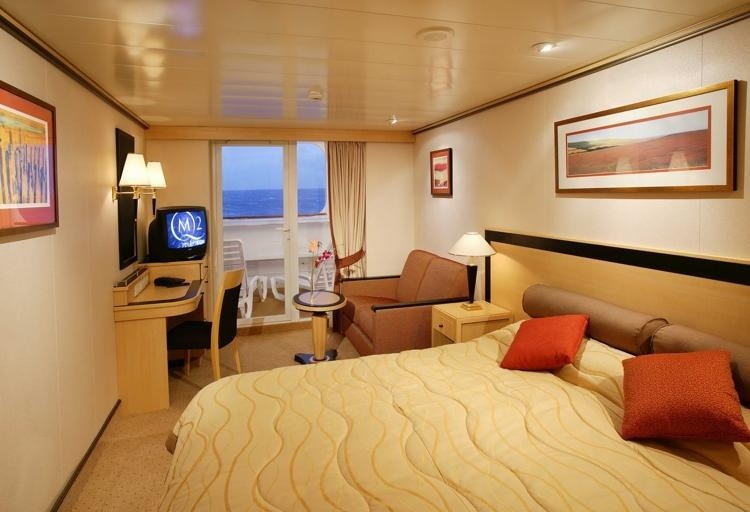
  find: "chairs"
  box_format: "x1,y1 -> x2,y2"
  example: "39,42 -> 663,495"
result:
167,267 -> 247,382
270,254 -> 337,302
222,239 -> 269,321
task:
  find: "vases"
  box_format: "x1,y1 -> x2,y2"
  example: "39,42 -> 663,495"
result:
311,265 -> 319,296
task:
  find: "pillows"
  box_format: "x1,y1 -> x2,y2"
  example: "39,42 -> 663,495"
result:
499,313 -> 592,371
521,280 -> 667,363
620,349 -> 750,443
652,321 -> 750,406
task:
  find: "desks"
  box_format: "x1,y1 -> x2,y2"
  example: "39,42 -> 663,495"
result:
114,279 -> 203,417
292,289 -> 348,364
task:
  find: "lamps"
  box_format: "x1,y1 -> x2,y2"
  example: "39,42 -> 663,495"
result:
111,152 -> 151,203
137,161 -> 167,200
447,232 -> 497,311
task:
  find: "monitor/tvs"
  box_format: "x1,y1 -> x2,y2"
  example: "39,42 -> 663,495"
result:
148,206 -> 208,262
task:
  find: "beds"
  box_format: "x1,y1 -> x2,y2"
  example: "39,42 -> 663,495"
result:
153,229 -> 750,512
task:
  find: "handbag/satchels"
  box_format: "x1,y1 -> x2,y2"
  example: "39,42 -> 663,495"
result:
154,278 -> 190,287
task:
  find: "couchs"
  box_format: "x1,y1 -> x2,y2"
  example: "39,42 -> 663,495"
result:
336,249 -> 471,357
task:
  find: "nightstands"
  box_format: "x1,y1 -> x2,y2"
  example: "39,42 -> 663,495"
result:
431,300 -> 513,347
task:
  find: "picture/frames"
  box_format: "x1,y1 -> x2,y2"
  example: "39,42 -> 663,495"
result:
554,80 -> 736,195
0,81 -> 60,236
430,148 -> 452,196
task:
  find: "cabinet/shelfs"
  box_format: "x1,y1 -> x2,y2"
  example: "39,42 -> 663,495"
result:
137,251 -> 209,358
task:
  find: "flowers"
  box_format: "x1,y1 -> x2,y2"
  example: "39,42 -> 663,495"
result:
306,238 -> 334,284
